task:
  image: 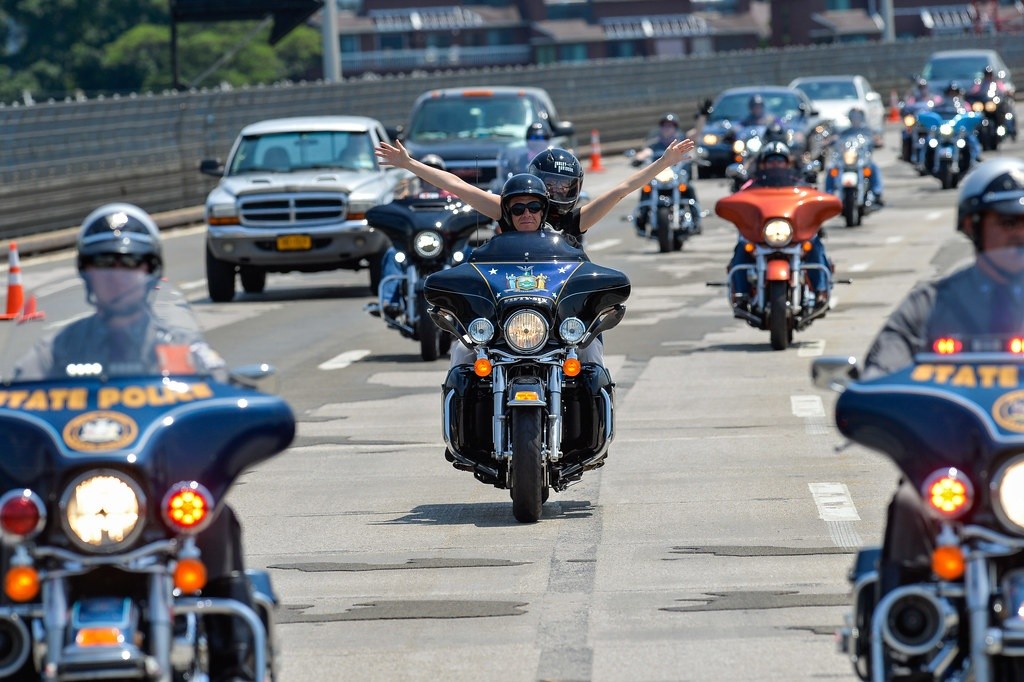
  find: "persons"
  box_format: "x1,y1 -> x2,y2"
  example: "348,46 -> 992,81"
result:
448,174 -> 604,370
859,152 -> 1024,601
630,65 -> 1017,316
363,153 -> 446,317
11,202 -> 227,380
508,121 -> 554,178
374,138 -> 695,252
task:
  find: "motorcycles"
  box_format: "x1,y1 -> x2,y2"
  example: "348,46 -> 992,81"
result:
631,145 -> 704,253
824,127 -> 885,227
915,100 -> 984,190
973,83 -> 1017,150
811,247 -> 1023,682
708,148 -> 843,353
732,119 -> 779,189
421,231 -> 632,526
894,92 -> 940,161
1,269 -> 296,682
365,180 -> 502,361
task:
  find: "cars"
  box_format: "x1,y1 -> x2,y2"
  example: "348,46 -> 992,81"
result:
697,87 -> 829,180
786,72 -> 885,139
910,50 -> 1014,114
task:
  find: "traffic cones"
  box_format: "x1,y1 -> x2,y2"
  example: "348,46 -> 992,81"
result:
0,239 -> 43,325
586,127 -> 607,176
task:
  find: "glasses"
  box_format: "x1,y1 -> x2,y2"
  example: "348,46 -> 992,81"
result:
78,252 -> 148,271
996,210 -> 1024,230
499,173 -> 550,235
509,201 -> 545,216
543,175 -> 573,188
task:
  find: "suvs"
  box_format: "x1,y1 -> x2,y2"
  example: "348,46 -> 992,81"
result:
387,88 -> 576,207
199,115 -> 395,299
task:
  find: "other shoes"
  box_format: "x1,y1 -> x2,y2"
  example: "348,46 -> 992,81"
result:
445,446 -> 455,463
817,291 -> 828,309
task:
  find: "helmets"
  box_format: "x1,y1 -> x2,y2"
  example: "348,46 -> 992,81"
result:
747,92 -> 866,163
529,148 -> 584,214
525,121 -> 553,154
956,155 -> 1024,233
657,113 -> 678,128
915,65 -> 998,100
75,203 -> 163,273
418,154 -> 446,191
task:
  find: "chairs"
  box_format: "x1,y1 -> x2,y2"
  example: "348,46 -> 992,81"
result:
262,147 -> 291,169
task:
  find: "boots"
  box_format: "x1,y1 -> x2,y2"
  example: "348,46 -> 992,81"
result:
201,571 -> 260,682
